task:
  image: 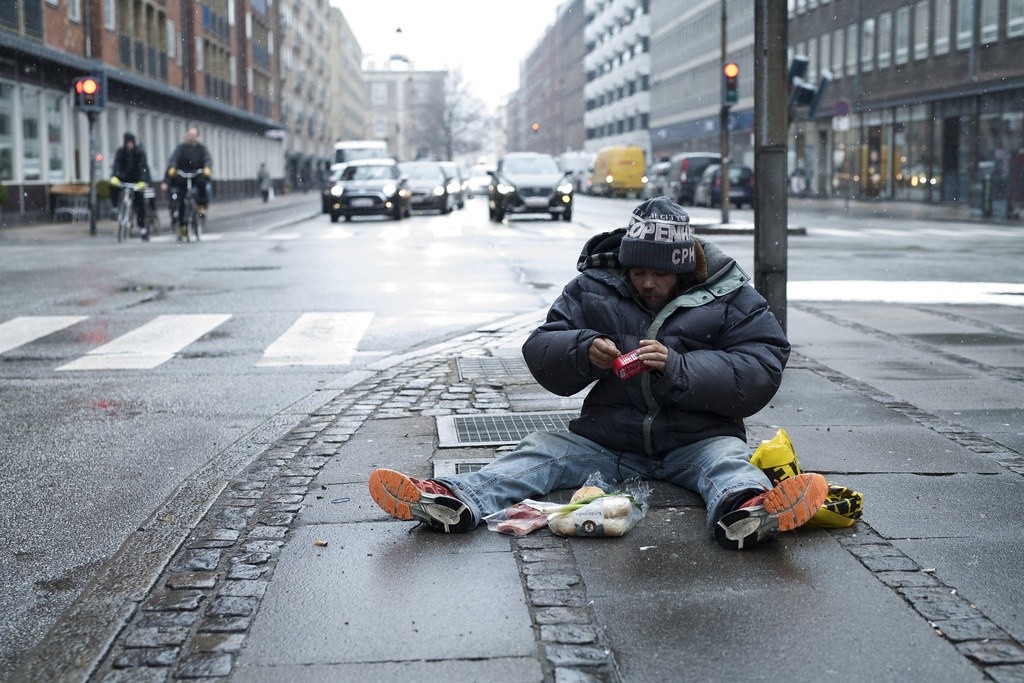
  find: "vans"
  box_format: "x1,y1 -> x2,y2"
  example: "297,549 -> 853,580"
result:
588,146 -> 645,199
320,162 -> 346,214
332,140 -> 387,167
663,153 -> 722,204
556,151 -> 591,179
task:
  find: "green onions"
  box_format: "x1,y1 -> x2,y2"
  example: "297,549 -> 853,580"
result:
540,490 -> 643,522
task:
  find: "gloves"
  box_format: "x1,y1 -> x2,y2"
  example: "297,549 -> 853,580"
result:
137,182 -> 145,190
204,167 -> 211,175
110,176 -> 120,187
168,168 -> 176,176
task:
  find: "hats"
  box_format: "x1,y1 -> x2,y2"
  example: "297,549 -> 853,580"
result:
125,133 -> 134,142
619,196 -> 697,273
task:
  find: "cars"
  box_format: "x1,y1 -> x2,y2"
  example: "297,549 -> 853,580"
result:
329,160 -> 413,223
396,161 -> 455,215
464,164 -> 497,200
486,151 -> 574,223
643,163 -> 669,200
575,164 -> 594,195
693,163 -> 758,210
438,162 -> 469,208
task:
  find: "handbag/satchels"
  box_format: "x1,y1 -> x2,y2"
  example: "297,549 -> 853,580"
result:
748,428 -> 864,526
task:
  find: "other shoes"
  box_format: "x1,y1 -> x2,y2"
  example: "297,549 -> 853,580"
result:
178,225 -> 185,236
197,205 -> 206,217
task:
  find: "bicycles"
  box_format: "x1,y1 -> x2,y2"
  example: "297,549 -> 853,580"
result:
112,180 -> 152,242
169,168 -> 210,243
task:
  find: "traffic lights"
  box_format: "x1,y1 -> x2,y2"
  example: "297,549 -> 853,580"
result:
532,122 -> 538,134
74,76 -> 103,113
726,63 -> 738,107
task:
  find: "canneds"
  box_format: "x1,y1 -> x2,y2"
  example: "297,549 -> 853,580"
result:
612,348 -> 655,381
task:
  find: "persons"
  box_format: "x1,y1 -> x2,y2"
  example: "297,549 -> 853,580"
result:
110,133 -> 155,240
369,194 -> 828,550
165,128 -> 213,235
258,163 -> 270,202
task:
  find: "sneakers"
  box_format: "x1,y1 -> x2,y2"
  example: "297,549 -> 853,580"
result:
715,473 -> 829,551
369,469 -> 473,535
